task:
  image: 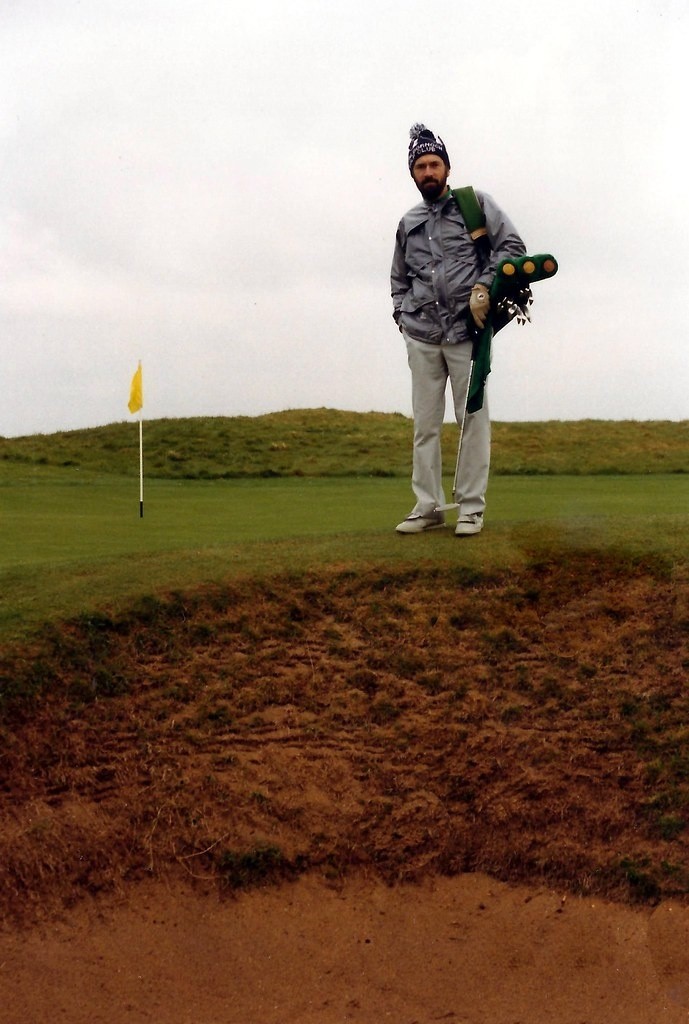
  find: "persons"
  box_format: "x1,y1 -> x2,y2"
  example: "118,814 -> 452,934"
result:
389,123 -> 527,536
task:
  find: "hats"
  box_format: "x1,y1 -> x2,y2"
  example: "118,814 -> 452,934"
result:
408,123 -> 450,178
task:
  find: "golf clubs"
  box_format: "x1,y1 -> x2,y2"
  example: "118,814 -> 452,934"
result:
432,318 -> 481,513
493,286 -> 534,327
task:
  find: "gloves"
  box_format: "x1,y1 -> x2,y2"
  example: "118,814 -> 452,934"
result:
469,284 -> 490,329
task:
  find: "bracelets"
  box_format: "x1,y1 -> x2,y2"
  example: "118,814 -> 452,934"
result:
470,283 -> 489,292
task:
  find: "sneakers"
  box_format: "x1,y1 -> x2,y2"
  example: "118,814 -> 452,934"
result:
396,513 -> 447,533
454,516 -> 485,535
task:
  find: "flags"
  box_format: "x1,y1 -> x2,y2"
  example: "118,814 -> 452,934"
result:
128,365 -> 143,415
